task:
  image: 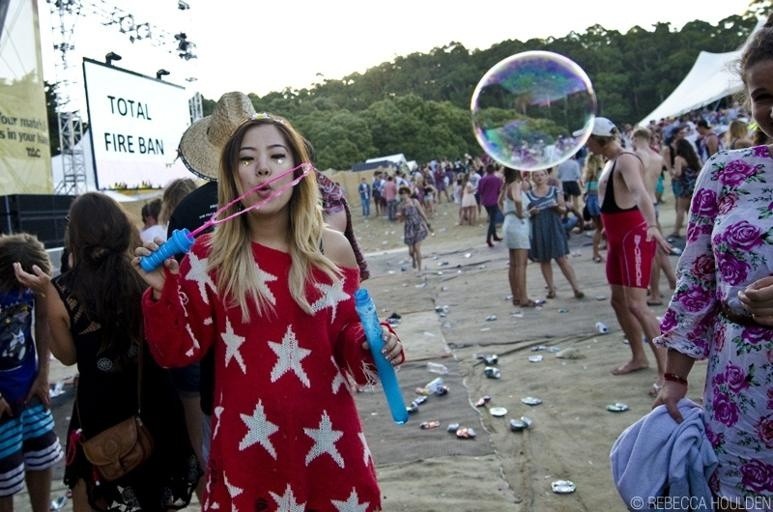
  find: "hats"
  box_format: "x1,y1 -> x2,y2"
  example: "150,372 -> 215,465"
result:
177,92 -> 257,181
573,118 -> 619,137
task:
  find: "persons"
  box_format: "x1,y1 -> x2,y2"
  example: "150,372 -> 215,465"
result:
653,16 -> 773,510
165,91 -> 371,470
357,95 -> 757,309
572,117 -> 675,398
302,135 -> 371,283
129,113 -> 404,512
0,176 -> 205,512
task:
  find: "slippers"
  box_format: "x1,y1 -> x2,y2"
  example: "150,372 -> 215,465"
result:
513,290 -> 583,307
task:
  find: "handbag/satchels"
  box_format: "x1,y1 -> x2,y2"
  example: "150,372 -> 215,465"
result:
82,417 -> 151,479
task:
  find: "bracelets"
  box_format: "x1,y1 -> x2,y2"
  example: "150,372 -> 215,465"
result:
0,393 -> 3,400
664,371 -> 687,386
